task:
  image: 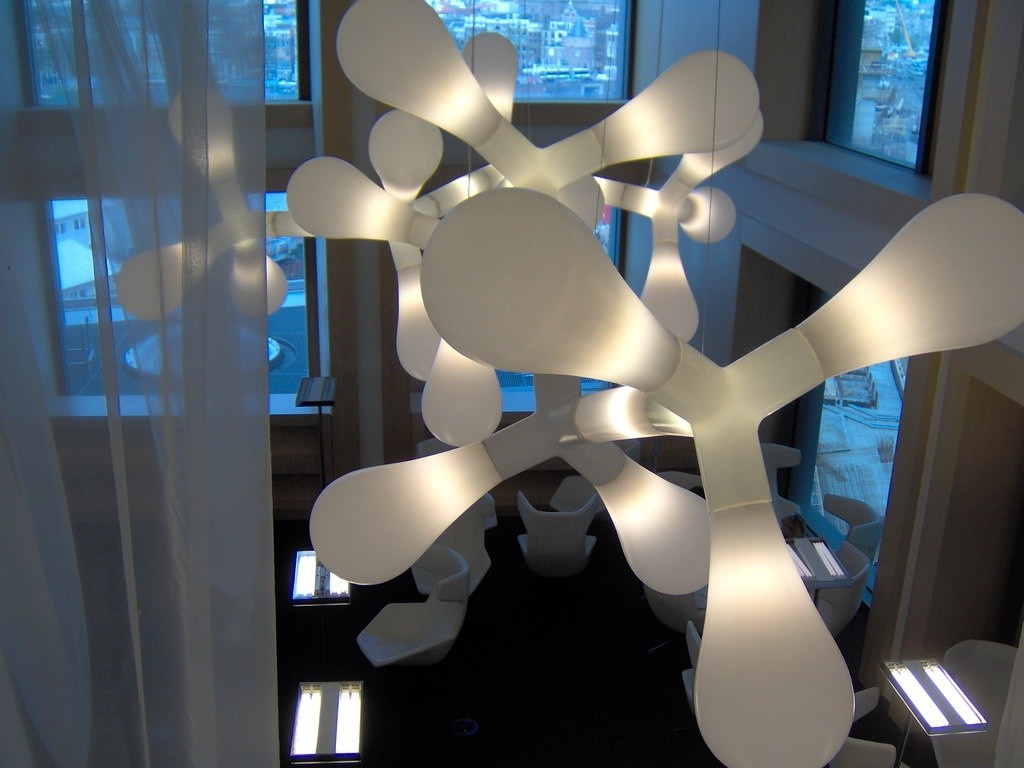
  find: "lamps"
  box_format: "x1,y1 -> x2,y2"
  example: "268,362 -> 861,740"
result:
112,0 -> 1024,768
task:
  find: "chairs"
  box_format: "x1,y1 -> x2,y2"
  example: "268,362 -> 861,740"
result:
825,493 -> 883,563
829,686 -> 911,768
761,441 -> 802,529
516,489 -> 599,577
409,494 -> 496,598
818,541 -> 872,638
548,475 -> 604,520
354,543 -> 472,667
682,620 -> 702,713
929,639 -> 1018,768
